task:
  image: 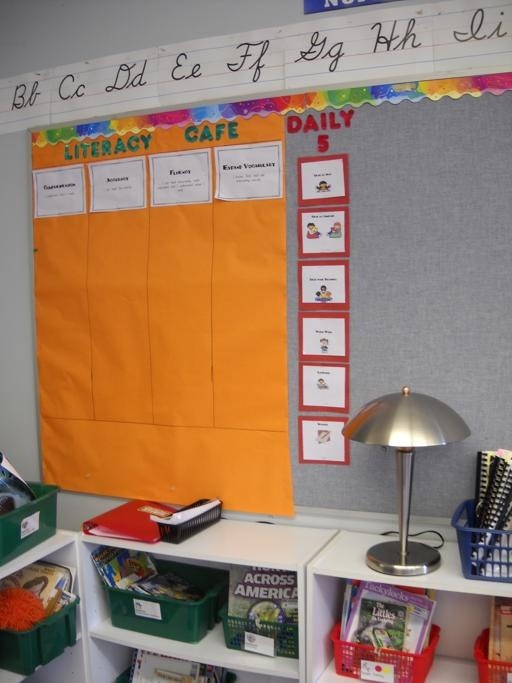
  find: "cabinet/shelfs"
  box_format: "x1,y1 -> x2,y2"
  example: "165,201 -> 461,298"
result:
79,518 -> 339,681
304,518 -> 511,682
0,529 -> 89,682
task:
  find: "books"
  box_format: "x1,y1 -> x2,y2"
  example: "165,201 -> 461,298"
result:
0,562 -> 77,628
337,582 -> 438,681
485,596 -> 511,666
464,447 -> 511,578
89,546 -> 205,603
227,564 -> 298,660
129,647 -> 227,682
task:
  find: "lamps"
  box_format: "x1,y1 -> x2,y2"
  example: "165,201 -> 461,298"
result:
340,383 -> 472,575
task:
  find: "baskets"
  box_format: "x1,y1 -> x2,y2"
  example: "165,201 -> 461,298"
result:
156,496 -> 223,543
450,496 -> 512,583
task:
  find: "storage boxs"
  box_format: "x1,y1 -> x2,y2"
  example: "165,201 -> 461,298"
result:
1,478 -> 61,567
103,565 -> 231,645
1,596 -> 80,677
